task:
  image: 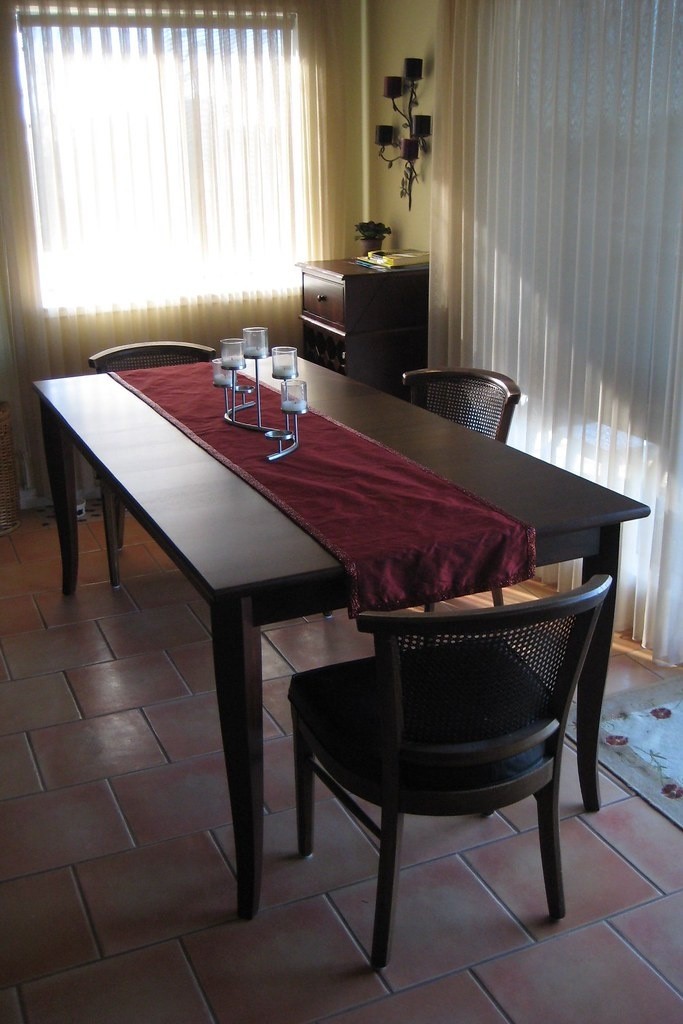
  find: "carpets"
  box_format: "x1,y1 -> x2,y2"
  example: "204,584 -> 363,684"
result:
563,671 -> 683,834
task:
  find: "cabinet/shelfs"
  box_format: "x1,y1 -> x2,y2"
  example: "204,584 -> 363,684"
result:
296,253 -> 431,405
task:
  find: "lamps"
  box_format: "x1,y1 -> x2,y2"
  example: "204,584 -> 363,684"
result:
375,58 -> 433,211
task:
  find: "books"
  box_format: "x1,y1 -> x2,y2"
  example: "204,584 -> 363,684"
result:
355,248 -> 429,272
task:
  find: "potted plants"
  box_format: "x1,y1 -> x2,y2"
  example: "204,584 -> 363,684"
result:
355,221 -> 391,257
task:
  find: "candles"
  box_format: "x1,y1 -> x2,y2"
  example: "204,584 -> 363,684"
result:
220,338 -> 246,370
281,379 -> 308,415
272,346 -> 299,380
243,327 -> 269,360
212,358 -> 238,388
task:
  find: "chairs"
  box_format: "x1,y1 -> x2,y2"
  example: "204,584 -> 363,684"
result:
88,339 -> 220,591
285,574 -> 615,969
320,368 -> 522,647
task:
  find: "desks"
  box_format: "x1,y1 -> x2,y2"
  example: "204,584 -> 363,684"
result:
32,351 -> 654,921
